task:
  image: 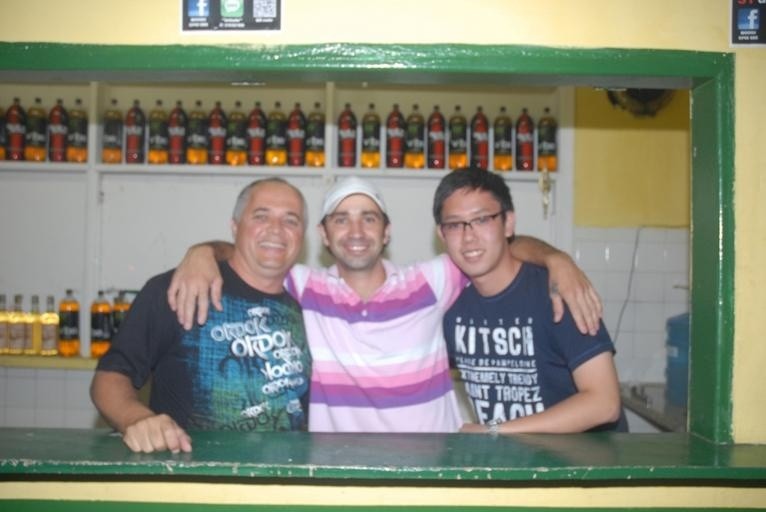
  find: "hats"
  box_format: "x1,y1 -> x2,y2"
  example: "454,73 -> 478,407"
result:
318,176 -> 389,223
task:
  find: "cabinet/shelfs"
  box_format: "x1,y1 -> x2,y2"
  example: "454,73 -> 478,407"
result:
2,69 -> 574,428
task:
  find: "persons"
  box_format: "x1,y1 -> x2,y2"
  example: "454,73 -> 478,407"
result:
433,166 -> 630,434
168,176 -> 602,433
90,177 -> 313,453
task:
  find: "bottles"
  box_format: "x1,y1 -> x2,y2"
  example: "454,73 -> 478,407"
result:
427,106 -> 445,169
102,100 -> 122,163
338,104 -> 357,167
470,106 -> 489,170
385,104 -> 405,167
168,101 -> 186,163
65,99 -> 88,162
7,295 -> 25,354
112,292 -> 132,336
246,101 -> 265,166
361,103 -> 380,166
208,102 -> 226,163
448,106 -> 468,170
24,295 -> 42,356
57,289 -> 81,357
404,104 -> 425,169
494,107 -> 512,171
49,99 -> 67,160
537,106 -> 558,173
0,295 -> 9,354
90,291 -> 112,358
306,101 -> 324,167
25,98 -> 46,161
185,101 -> 208,163
5,97 -> 26,161
149,101 -> 168,163
287,103 -> 305,166
265,102 -> 287,167
225,101 -> 246,166
126,100 -> 146,164
40,296 -> 60,357
514,107 -> 535,171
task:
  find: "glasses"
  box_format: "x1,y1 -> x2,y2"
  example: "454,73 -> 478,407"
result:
439,209 -> 506,238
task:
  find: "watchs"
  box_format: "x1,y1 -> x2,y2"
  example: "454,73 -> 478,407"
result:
488,417 -> 502,434
